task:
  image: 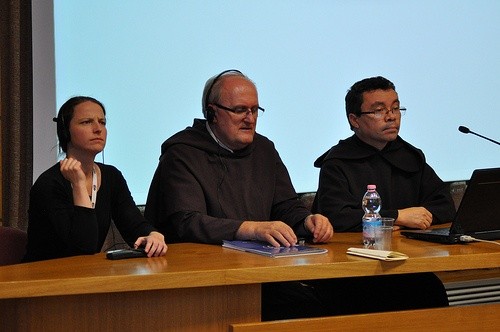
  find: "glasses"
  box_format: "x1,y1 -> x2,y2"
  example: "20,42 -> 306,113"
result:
361,106 -> 407,117
207,101 -> 265,117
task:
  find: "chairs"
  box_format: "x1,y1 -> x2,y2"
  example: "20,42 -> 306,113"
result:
300,193 -> 317,214
449,180 -> 470,215
1,226 -> 34,266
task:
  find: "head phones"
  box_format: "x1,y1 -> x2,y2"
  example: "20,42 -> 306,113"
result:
58,103 -> 71,142
205,70 -> 243,125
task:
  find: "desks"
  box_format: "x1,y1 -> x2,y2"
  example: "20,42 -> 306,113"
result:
0,221 -> 500,332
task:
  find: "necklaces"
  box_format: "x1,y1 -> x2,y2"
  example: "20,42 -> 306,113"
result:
71,165 -> 97,209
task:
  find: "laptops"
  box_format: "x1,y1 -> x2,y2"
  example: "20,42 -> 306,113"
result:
401,168 -> 500,243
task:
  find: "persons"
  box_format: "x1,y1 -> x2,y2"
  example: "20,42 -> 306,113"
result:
143,69 -> 333,323
310,76 -> 457,314
24,96 -> 168,257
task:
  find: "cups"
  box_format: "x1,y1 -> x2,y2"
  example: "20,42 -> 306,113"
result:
374,226 -> 393,250
382,217 -> 395,226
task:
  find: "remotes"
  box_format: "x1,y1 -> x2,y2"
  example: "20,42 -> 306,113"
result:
106,249 -> 148,260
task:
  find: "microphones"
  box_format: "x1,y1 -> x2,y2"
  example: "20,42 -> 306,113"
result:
458,126 -> 500,146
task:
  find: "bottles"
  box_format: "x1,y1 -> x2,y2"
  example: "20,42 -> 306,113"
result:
362,185 -> 382,248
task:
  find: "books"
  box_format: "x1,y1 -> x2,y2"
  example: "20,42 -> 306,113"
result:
222,239 -> 328,259
